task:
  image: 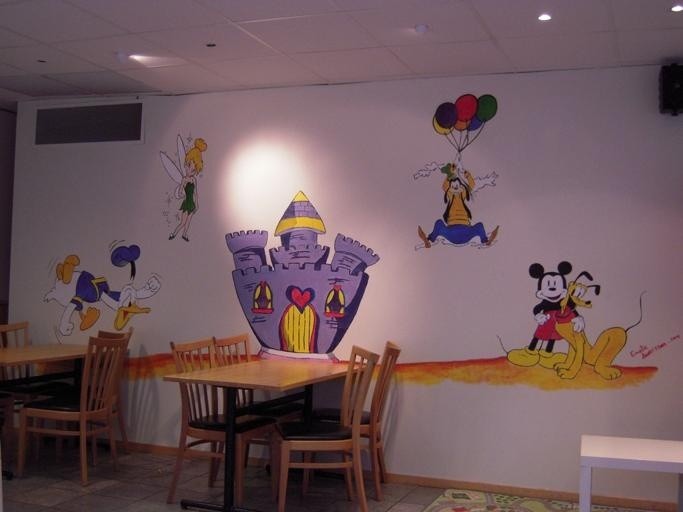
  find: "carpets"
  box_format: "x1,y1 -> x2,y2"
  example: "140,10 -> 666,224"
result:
422,488 -> 639,512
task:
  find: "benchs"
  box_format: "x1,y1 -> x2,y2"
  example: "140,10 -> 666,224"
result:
579,435 -> 682,511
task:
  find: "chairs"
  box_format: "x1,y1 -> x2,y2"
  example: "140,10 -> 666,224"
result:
297,338 -> 400,502
1,320 -> 134,487
263,344 -> 382,506
167,336 -> 280,507
211,332 -> 320,491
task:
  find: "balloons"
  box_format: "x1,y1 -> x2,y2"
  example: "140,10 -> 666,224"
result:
433,94 -> 498,152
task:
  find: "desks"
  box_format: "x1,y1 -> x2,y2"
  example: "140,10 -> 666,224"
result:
162,353 -> 375,510
